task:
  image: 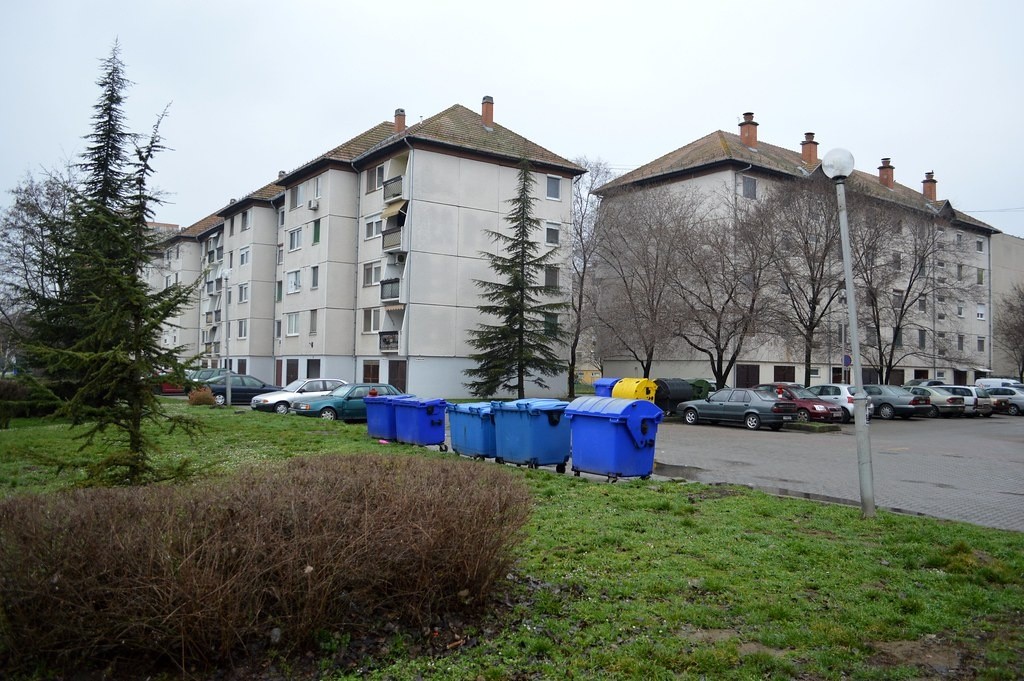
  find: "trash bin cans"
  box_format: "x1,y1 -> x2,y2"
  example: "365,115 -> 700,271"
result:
563,395 -> 665,484
391,396 -> 449,453
593,376 -> 621,397
612,377 -> 659,404
362,393 -> 416,441
652,376 -> 693,416
445,400 -> 496,461
490,396 -> 572,473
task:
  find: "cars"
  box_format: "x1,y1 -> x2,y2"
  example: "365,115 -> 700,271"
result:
750,382 -> 844,424
862,384 -> 933,420
676,387 -> 798,431
191,374 -> 285,405
250,379 -> 365,415
290,383 -> 402,423
984,386 -> 1024,416
900,379 -> 1011,418
705,379 -> 731,397
139,367 -> 238,394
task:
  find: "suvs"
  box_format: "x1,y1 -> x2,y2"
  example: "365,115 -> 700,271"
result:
803,384 -> 874,423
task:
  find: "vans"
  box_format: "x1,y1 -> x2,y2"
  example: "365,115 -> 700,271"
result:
975,378 -> 1024,389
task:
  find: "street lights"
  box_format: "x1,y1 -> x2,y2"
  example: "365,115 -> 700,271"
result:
221,268 -> 232,407
823,146 -> 877,518
